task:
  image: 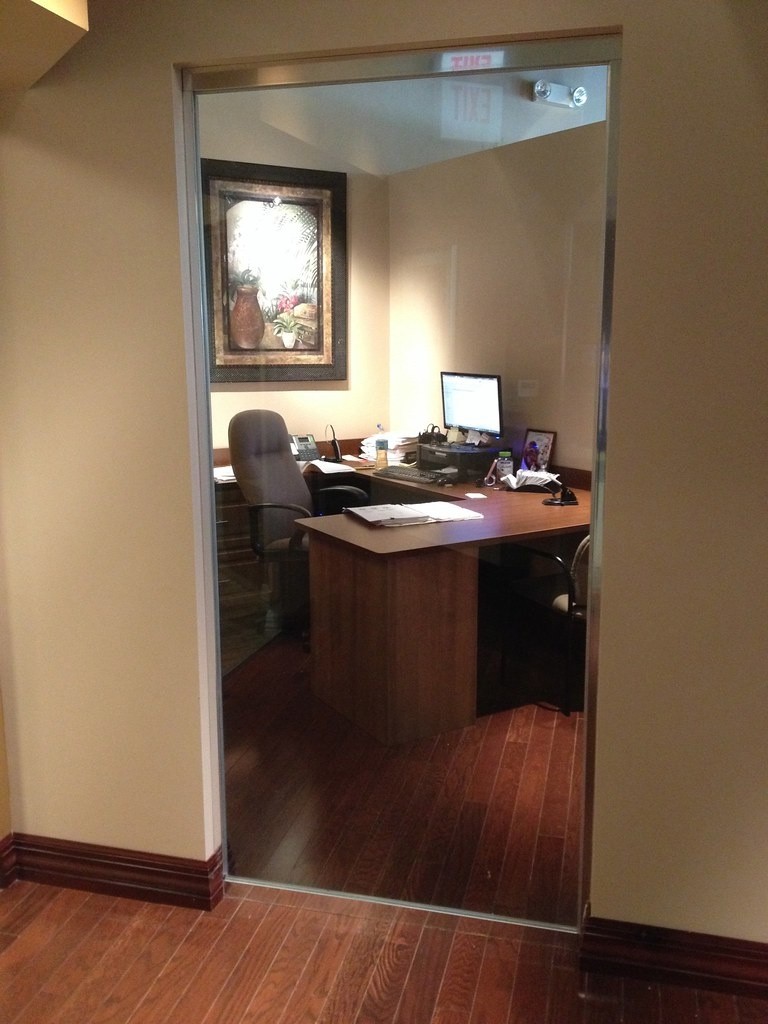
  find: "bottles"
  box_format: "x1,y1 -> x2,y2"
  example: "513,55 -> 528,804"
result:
497,452 -> 514,482
375,429 -> 389,473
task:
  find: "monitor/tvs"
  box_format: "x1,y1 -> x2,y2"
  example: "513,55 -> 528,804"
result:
441,371 -> 504,448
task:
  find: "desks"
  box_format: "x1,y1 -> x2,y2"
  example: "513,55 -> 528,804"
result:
215,451 -> 593,755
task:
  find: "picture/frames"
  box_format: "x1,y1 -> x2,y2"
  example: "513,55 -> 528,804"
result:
202,157 -> 348,383
521,427 -> 558,472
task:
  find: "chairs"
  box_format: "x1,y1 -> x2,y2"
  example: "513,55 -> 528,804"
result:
229,409 -> 372,645
501,531 -> 590,693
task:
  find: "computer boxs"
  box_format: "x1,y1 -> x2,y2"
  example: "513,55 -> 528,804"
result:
415,443 -> 513,482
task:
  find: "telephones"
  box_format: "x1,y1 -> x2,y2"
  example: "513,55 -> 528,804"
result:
289,433 -> 321,461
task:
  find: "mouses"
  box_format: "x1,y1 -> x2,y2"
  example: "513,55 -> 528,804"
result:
437,476 -> 458,485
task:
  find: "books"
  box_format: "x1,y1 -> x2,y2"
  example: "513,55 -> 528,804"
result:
296,459 -> 357,475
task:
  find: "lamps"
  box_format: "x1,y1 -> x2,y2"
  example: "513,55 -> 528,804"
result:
532,79 -> 588,109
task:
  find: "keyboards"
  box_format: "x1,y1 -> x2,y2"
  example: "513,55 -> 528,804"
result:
372,465 -> 448,484
